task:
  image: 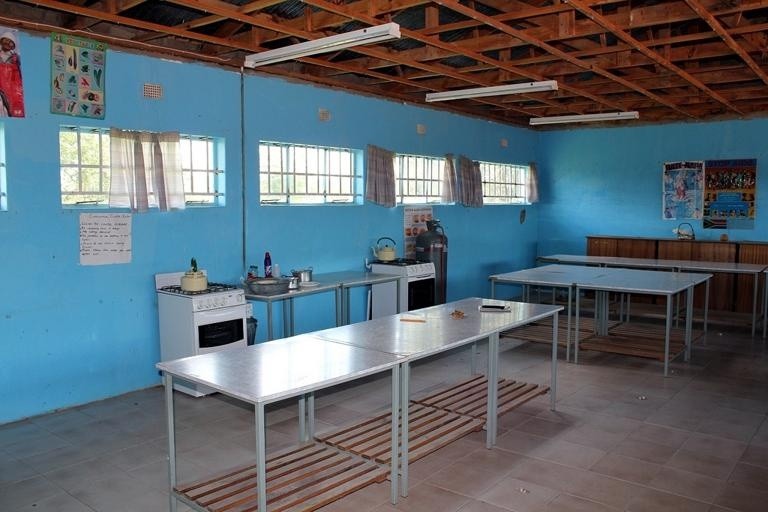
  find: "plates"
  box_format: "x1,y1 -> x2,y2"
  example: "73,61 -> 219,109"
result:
297,282 -> 320,288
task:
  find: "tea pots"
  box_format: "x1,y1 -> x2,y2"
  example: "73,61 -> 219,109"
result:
180,260 -> 208,294
370,237 -> 397,263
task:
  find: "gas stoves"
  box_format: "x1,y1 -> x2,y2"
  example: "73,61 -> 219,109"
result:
158,282 -> 247,309
370,260 -> 436,277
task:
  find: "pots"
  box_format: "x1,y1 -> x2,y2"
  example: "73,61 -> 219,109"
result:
290,266 -> 313,282
283,275 -> 298,290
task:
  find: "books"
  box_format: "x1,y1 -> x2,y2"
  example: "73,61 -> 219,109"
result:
478,299 -> 511,312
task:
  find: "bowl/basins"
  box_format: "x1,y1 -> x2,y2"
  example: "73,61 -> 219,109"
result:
248,278 -> 290,295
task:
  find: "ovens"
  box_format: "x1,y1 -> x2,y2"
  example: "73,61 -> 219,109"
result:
158,312 -> 253,399
400,278 -> 438,313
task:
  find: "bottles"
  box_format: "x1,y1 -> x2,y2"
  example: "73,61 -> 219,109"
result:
264,252 -> 273,278
274,264 -> 280,278
247,265 -> 258,283
719,232 -> 728,242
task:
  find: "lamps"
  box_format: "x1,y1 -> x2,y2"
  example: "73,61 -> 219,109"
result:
528,110 -> 640,125
244,22 -> 401,70
426,78 -> 559,102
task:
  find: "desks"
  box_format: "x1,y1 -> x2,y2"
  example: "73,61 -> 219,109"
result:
307,312 -> 495,500
157,333 -> 408,511
408,296 -> 566,443
487,253 -> 767,377
311,270 -> 405,325
244,280 -> 343,343
